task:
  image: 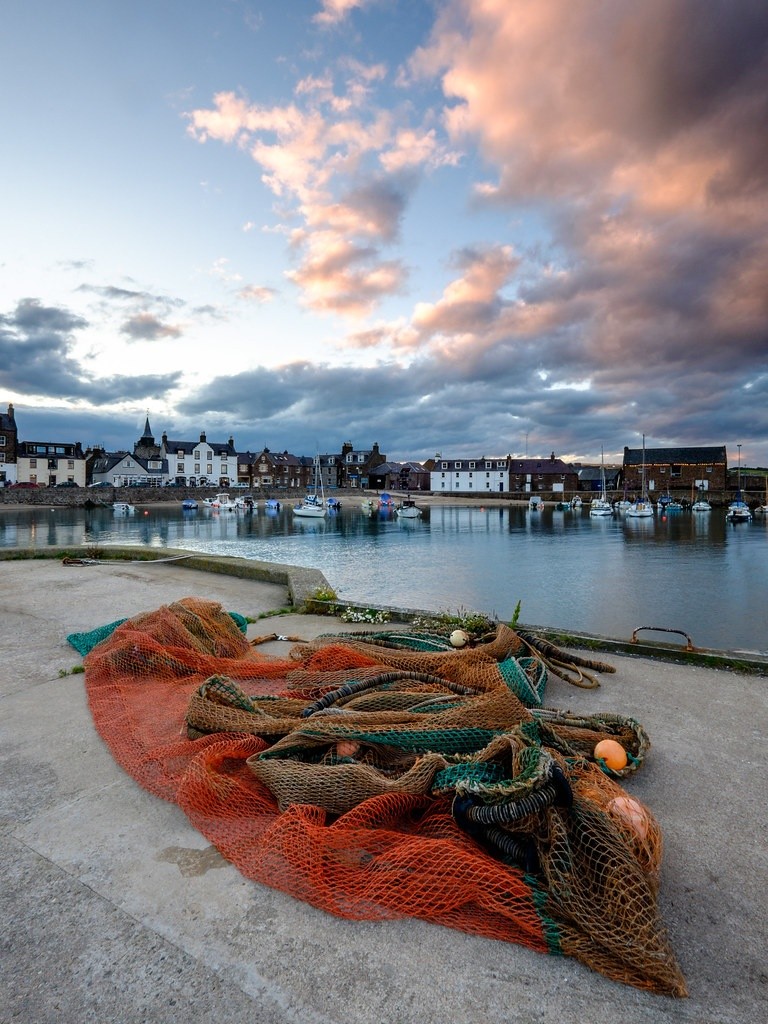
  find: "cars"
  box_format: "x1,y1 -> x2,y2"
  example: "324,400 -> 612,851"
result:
8,480 -> 251,487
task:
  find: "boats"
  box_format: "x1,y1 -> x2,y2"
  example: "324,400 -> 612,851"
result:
554,435 -> 767,519
293,448 -> 343,517
393,494 -> 423,518
113,504 -> 135,512
237,495 -> 258,509
203,492 -> 237,509
361,500 -> 378,517
379,492 -> 394,505
181,498 -> 199,509
529,497 -> 545,510
265,500 -> 284,509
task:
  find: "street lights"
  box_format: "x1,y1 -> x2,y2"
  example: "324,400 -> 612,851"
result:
737,444 -> 742,489
